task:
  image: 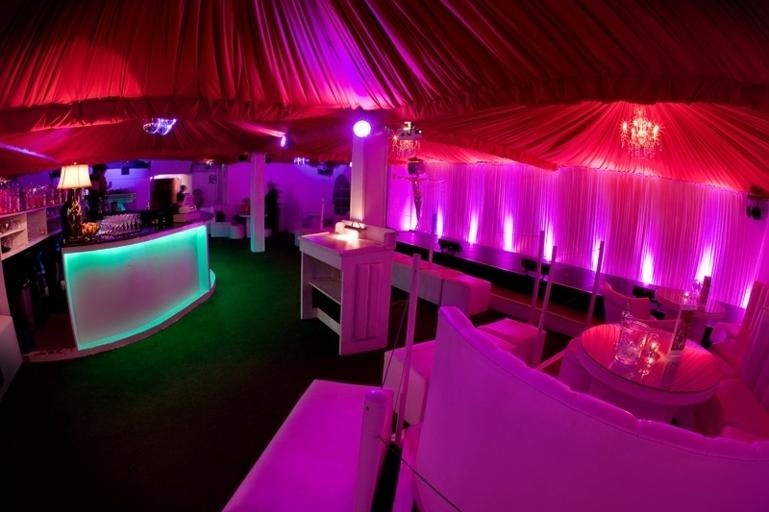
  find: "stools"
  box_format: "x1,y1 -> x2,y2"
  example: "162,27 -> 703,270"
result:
229,223 -> 244,239
382,317 -> 547,425
392,252 -> 492,317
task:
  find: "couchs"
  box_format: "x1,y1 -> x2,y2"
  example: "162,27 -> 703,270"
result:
393,282 -> 769,512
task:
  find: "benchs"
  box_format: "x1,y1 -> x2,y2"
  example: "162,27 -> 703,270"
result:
216,380 -> 398,512
211,204 -> 231,237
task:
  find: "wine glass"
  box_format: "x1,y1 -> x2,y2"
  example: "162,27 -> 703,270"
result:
96,213 -> 142,237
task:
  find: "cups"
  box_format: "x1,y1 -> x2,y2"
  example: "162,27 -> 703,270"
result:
612,319 -> 651,367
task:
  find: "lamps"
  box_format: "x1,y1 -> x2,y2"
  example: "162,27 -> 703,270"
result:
58,162 -> 93,244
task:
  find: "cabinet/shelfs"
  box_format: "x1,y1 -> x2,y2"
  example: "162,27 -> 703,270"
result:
0,202 -> 62,262
299,220 -> 396,356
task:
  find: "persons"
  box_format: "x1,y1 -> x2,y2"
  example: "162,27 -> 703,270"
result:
176,185 -> 186,201
59,163 -> 112,236
265,180 -> 280,237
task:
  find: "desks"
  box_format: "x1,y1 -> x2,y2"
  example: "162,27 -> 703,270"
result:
240,215 -> 269,238
655,287 -> 728,330
575,323 -> 725,423
